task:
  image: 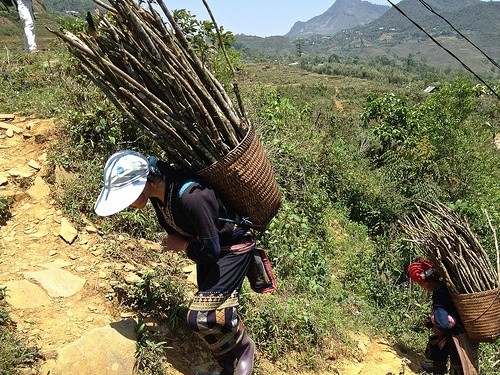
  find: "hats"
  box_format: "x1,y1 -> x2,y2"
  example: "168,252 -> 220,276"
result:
407,260 -> 441,283
94,149 -> 159,217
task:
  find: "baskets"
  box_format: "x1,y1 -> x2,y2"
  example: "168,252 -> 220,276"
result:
190,116 -> 283,233
450,286 -> 500,345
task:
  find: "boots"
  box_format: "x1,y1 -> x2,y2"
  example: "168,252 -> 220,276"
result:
219,363 -> 234,375
224,328 -> 256,375
421,359 -> 447,373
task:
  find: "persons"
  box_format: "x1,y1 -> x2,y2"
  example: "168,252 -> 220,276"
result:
93,150 -> 276,375
408,258 -> 481,375
